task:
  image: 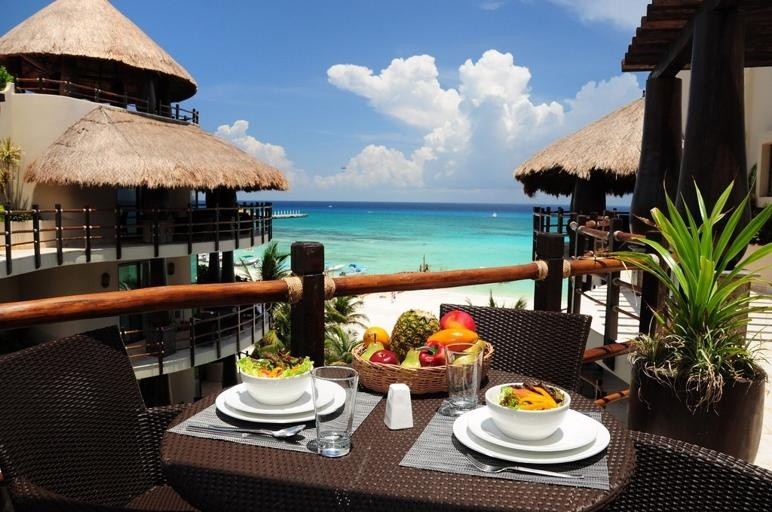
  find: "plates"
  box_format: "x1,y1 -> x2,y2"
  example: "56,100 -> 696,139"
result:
223,387 -> 334,416
452,405 -> 612,465
214,380 -> 348,424
466,409 -> 598,452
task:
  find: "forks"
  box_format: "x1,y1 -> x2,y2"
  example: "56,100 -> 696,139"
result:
463,453 -> 584,482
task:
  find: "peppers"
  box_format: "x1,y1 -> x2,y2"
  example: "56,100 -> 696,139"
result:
416,341 -> 445,367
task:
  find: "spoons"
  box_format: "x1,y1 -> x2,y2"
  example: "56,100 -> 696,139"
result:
187,421 -> 306,439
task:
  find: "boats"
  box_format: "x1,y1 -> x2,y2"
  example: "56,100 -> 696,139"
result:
198,251 -> 367,279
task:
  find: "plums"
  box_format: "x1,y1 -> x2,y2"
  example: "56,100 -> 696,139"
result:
360,333 -> 386,361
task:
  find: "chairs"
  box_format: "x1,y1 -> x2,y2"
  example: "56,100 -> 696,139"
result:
0,321 -> 200,512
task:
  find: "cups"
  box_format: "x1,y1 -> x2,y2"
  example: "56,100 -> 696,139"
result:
444,342 -> 484,417
309,365 -> 359,458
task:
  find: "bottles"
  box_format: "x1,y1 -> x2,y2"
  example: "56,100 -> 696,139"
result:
383,382 -> 415,429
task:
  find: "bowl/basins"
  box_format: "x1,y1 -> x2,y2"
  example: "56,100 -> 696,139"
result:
238,366 -> 314,405
482,383 -> 573,442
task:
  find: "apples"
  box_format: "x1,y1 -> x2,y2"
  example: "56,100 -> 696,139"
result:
369,349 -> 400,365
439,310 -> 475,332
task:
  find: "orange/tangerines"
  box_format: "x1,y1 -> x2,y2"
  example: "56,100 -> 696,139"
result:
363,326 -> 390,349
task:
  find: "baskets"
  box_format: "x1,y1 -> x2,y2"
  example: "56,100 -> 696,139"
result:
349,339 -> 494,397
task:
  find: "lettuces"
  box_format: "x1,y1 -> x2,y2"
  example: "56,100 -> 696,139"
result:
236,351 -> 314,377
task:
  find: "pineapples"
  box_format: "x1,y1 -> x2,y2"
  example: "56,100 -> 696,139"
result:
389,309 -> 440,362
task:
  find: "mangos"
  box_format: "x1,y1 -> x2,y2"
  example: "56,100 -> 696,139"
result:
426,328 -> 480,353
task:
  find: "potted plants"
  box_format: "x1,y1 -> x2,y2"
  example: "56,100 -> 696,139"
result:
599,170 -> 772,463
